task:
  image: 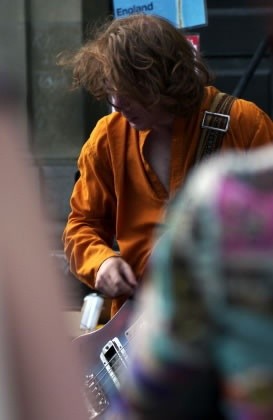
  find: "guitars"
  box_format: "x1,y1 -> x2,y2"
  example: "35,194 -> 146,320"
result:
65,297 -> 178,420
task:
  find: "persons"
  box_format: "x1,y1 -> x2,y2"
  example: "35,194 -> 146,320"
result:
61,13 -> 273,320
100,143 -> 273,420
